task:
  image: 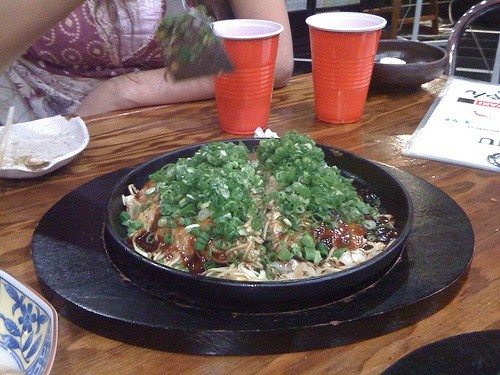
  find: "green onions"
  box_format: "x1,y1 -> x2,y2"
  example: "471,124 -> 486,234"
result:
118,127 -> 384,278
155,4 -> 219,76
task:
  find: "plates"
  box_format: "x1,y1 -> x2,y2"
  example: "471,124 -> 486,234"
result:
379,329 -> 500,375
0,115 -> 90,178
104,138 -> 414,309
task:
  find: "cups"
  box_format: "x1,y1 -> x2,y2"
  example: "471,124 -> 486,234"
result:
305,11 -> 387,124
209,19 -> 284,135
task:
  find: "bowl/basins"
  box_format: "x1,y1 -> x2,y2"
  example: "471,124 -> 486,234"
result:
367,39 -> 448,92
0,265 -> 59,375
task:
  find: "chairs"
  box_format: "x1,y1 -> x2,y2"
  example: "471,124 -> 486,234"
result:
442,0 -> 500,80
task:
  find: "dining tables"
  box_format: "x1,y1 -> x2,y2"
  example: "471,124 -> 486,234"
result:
0,61 -> 500,375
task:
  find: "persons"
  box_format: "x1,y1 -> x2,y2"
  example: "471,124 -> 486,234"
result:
1,0 -> 295,126
